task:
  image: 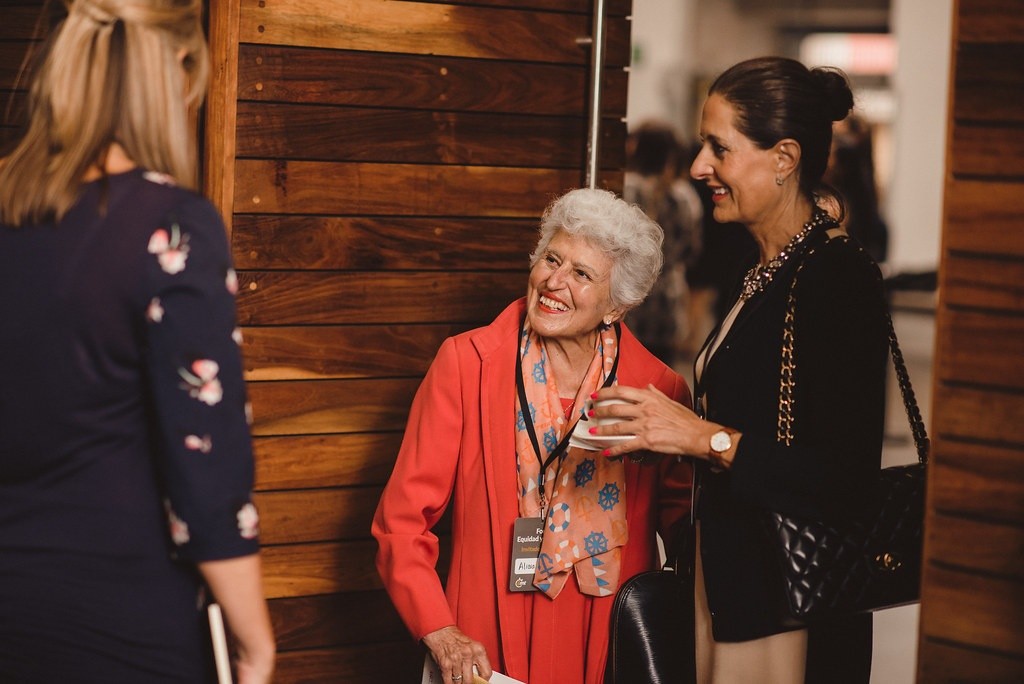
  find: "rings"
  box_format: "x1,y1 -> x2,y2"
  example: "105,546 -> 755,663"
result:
452,675 -> 463,680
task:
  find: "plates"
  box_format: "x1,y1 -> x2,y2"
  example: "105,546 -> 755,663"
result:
573,435 -> 635,449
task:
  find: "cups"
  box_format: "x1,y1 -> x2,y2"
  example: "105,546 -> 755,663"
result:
583,398 -> 633,426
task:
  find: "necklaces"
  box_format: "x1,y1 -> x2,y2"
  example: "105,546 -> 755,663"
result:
740,208 -> 833,303
562,399 -> 576,415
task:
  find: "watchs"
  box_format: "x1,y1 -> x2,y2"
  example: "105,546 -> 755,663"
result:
707,426 -> 740,467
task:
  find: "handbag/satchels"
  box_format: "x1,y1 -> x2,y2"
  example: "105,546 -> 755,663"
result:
700,236 -> 929,644
604,512 -> 873,684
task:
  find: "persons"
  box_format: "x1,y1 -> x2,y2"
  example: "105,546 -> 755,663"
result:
586,52 -> 895,684
370,191 -> 687,684
0,0 -> 282,684
